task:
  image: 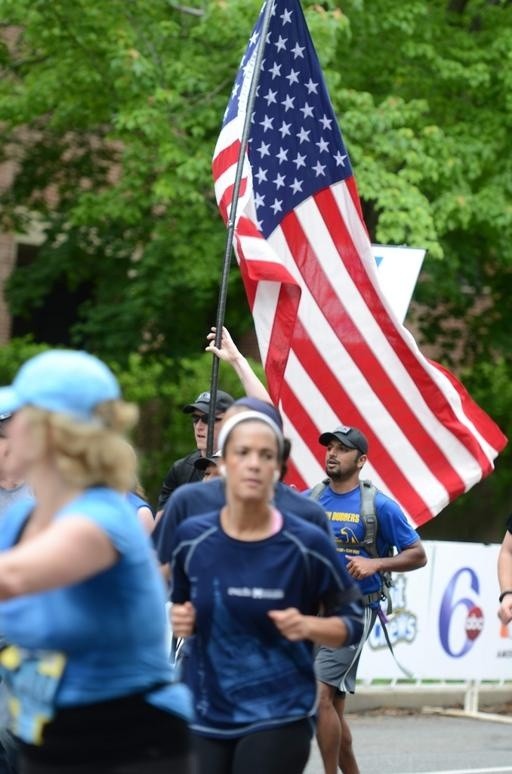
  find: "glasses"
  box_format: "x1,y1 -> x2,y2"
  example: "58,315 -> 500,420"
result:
191,414 -> 222,425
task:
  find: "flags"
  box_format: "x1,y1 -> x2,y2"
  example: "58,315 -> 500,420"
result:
212,0 -> 510,531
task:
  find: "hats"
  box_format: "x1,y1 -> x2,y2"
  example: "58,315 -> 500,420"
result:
0,349 -> 120,426
183,390 -> 233,415
319,427 -> 367,453
194,454 -> 220,468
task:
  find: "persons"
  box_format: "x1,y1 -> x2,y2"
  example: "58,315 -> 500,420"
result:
497,523 -> 512,625
299,423 -> 427,774
167,410 -> 364,774
126,325 -> 333,590
0,349 -> 196,773
0,386 -> 31,513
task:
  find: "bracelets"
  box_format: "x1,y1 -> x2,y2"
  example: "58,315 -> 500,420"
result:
499,591 -> 512,602
